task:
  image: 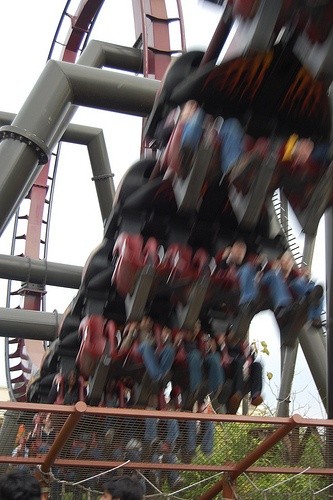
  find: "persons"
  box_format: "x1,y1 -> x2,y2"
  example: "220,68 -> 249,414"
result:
273,251 -> 324,328
130,314 -> 176,391
214,329 -> 263,410
0,469 -> 41,500
10,371 -> 215,489
100,476 -> 145,500
152,99 -> 260,186
173,326 -> 222,404
219,241 -> 295,326
